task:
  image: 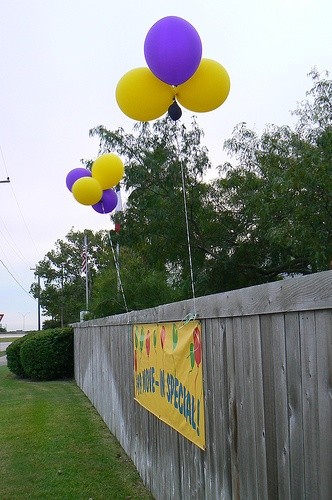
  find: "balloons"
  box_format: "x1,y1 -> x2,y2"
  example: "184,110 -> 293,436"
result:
144,16 -> 202,86
175,58 -> 230,121
66,153 -> 124,213
116,66 -> 176,122
